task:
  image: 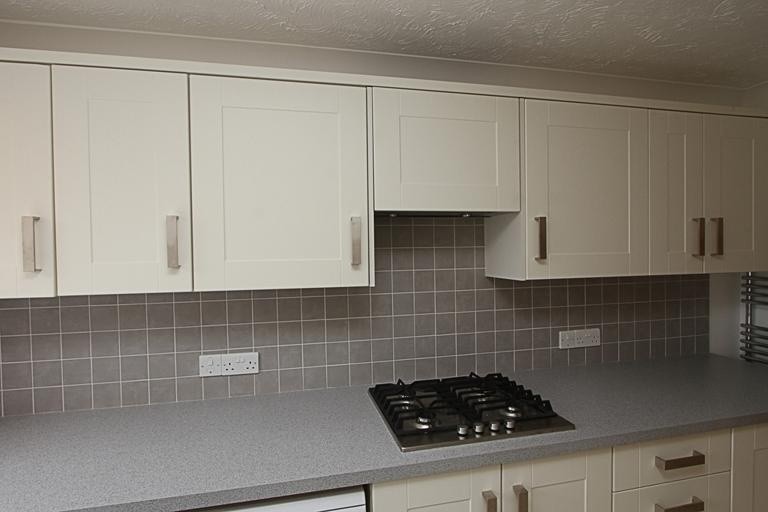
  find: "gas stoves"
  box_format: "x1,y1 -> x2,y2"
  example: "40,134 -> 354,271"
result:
368,371 -> 576,453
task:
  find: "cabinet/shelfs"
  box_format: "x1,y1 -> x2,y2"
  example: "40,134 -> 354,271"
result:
184,60 -> 382,292
650,101 -> 764,276
368,445 -> 611,511
479,84 -> 651,282
368,76 -> 526,222
0,47 -> 54,298
611,423 -> 734,510
730,419 -> 767,510
49,49 -> 195,296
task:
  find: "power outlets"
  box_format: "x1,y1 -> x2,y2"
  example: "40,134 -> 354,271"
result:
222,350 -> 261,376
575,328 -> 601,347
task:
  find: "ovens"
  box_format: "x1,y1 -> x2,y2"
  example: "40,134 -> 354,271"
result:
209,487 -> 367,510
